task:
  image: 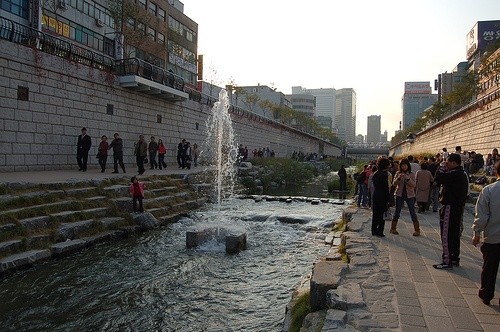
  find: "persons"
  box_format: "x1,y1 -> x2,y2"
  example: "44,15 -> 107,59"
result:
338,165 -> 347,200
237,144 -> 248,162
292,150 -> 317,162
406,132 -> 414,140
177,139 -> 192,170
254,147 -> 275,158
352,145 -> 500,307
157,138 -> 167,171
133,134 -> 148,176
168,70 -> 175,86
109,133 -> 127,174
192,144 -> 198,167
77,127 -> 92,172
320,154 -> 326,161
130,176 -> 146,215
342,151 -> 345,157
371,158 -> 389,237
433,153 -> 468,270
144,60 -> 152,77
147,136 -> 158,170
389,159 -> 420,236
97,136 -> 111,173
471,162 -> 500,307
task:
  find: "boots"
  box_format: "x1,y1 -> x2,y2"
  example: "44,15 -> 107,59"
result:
376,221 -> 386,237
390,221 -> 398,234
413,222 -> 420,236
372,222 -> 377,235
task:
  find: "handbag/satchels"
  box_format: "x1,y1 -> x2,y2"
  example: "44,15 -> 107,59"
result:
96,152 -> 101,158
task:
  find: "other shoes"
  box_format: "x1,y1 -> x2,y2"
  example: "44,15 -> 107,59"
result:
79,168 -> 83,171
433,263 -> 453,269
483,300 -> 490,306
83,169 -> 86,172
451,261 -> 460,266
112,170 -> 118,173
